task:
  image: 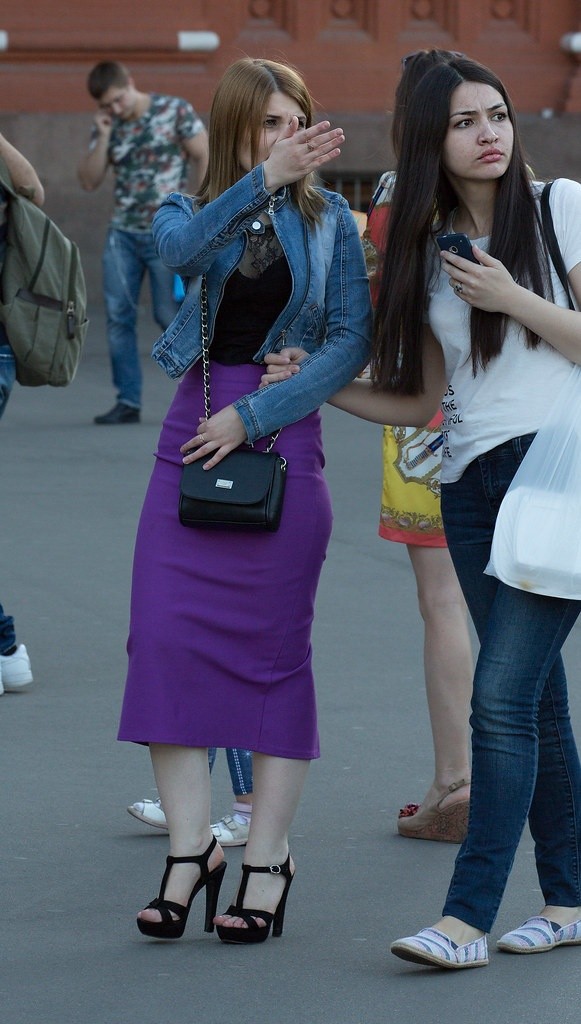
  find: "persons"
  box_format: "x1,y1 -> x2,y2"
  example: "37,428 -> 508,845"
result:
77,61 -> 215,426
341,45 -> 499,843
131,721 -> 271,846
136,54 -> 381,946
259,59 -> 581,973
0,127 -> 52,693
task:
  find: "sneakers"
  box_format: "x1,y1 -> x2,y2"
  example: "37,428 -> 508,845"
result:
0,644 -> 33,696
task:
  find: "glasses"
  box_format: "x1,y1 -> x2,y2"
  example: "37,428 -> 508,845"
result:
402,53 -> 415,69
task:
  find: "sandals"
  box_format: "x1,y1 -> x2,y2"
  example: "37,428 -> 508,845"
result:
210,815 -> 250,847
127,799 -> 169,829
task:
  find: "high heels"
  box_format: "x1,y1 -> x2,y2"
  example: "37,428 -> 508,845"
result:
137,835 -> 227,939
216,852 -> 296,944
398,780 -> 471,844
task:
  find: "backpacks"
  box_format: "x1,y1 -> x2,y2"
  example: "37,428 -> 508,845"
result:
0,158 -> 89,388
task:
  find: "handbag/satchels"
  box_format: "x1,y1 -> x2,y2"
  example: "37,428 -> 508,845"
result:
483,363 -> 580,600
179,448 -> 286,533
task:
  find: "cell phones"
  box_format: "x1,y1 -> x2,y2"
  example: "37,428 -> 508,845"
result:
438,234 -> 480,273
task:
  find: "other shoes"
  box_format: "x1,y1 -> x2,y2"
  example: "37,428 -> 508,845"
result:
495,917 -> 580,953
389,927 -> 490,967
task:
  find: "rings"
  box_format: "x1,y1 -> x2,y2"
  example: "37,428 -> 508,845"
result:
198,434 -> 207,444
306,140 -> 315,152
454,282 -> 463,293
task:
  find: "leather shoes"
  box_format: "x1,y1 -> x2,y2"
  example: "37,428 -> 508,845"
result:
94,403 -> 141,423
